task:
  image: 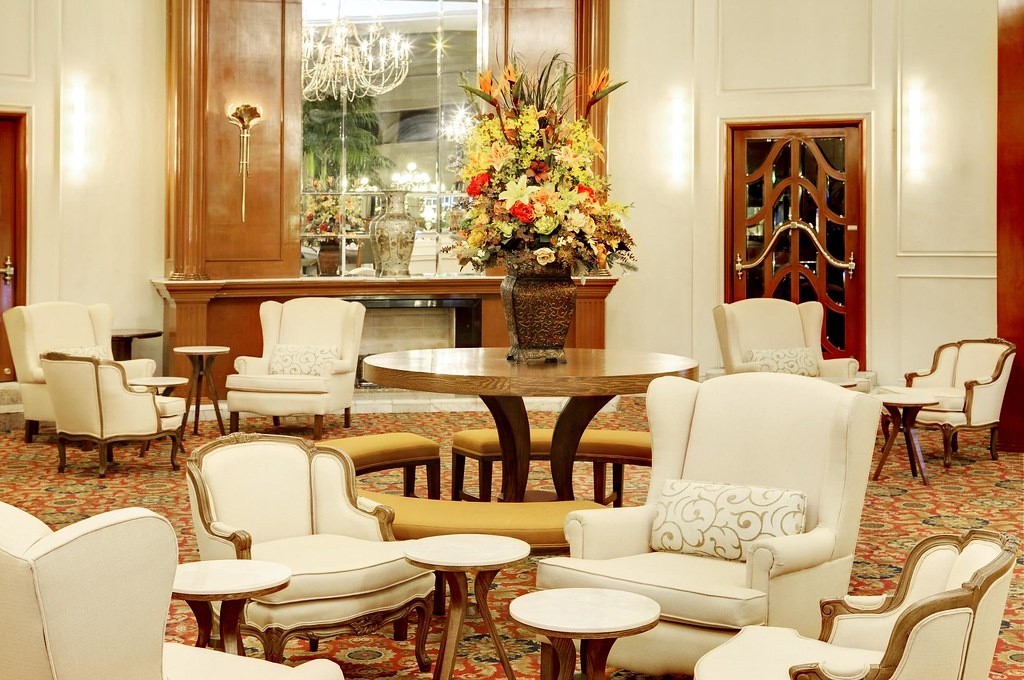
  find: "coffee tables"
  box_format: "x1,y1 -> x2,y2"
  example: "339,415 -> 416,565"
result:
404,533 -> 530,680
509,588 -> 661,680
127,377 -> 189,457
173,345 -> 230,440
170,559 -> 292,657
874,394 -> 940,486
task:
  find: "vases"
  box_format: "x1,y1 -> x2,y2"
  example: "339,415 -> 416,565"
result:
374,190 -> 417,278
500,255 -> 577,365
316,240 -> 339,276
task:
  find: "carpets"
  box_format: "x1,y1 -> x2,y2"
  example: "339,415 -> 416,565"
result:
0,412 -> 1024,680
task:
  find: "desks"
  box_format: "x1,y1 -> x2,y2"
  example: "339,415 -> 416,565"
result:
362,348 -> 699,555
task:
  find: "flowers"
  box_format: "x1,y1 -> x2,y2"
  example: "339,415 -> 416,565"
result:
307,190 -> 363,244
441,41 -> 636,286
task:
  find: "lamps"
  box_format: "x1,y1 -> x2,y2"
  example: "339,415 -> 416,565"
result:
302,1 -> 411,102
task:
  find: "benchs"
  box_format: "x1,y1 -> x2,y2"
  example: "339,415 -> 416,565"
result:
311,429 -> 652,613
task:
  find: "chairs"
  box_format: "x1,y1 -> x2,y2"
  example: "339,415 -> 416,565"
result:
694,529 -> 1021,680
186,431 -> 435,672
226,297 -> 366,439
714,298 -> 871,395
904,338 -> 1016,467
3,302 -> 156,451
0,499 -> 344,680
39,353 -> 186,477
536,372 -> 883,676
